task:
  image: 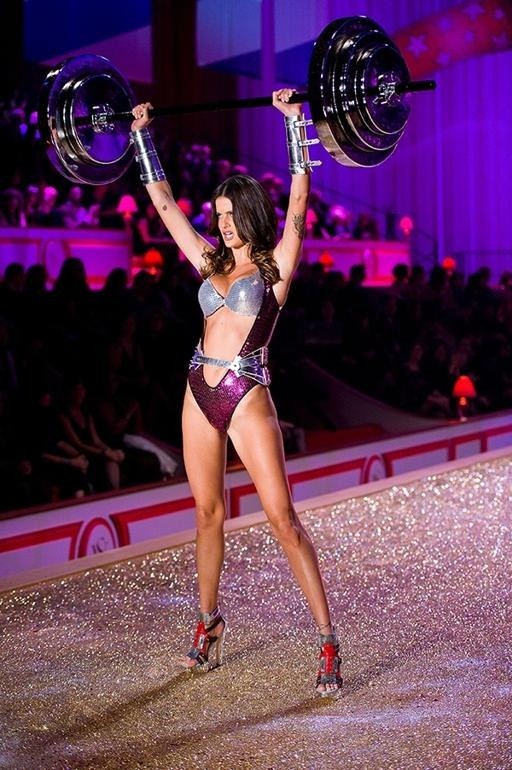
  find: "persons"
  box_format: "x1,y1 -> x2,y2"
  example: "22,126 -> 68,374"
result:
130,87 -> 344,694
0,95 -> 411,276
2,257 -> 512,511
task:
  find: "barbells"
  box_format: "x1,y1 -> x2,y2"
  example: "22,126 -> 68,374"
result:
37,16 -> 436,186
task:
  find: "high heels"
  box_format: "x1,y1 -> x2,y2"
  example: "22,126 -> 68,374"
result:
186,607 -> 226,678
313,627 -> 343,699
